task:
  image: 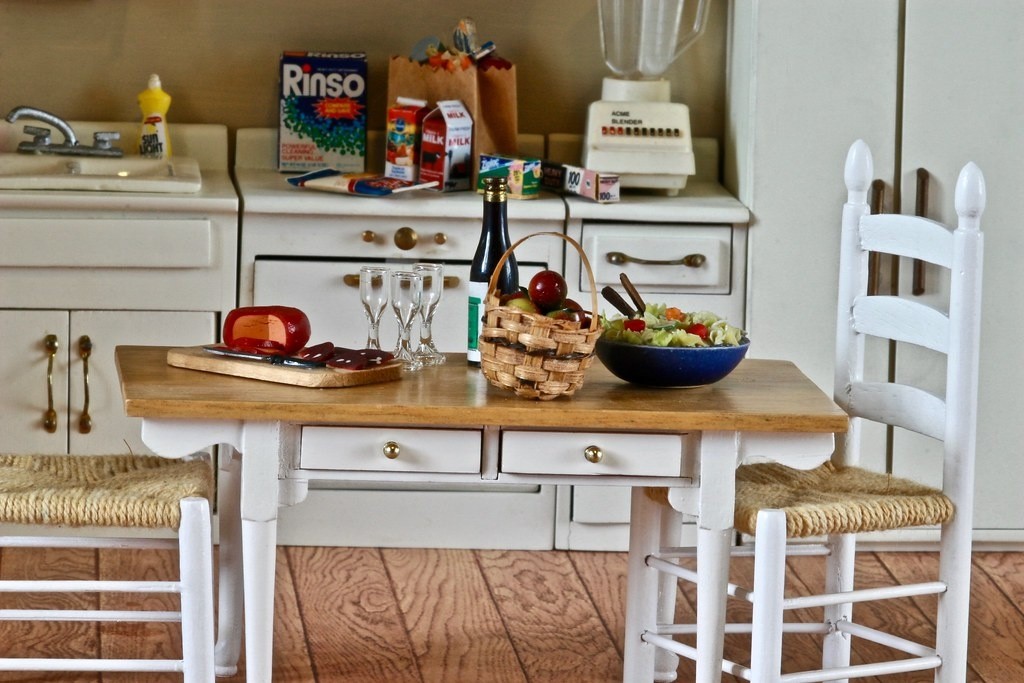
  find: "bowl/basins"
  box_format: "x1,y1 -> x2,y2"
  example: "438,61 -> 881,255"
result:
596,334 -> 751,389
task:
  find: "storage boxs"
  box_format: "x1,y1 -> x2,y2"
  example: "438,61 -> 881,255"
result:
475,153 -> 542,199
544,158 -> 621,205
277,48 -> 366,174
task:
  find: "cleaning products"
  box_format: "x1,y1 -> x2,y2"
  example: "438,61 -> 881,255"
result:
135,72 -> 173,160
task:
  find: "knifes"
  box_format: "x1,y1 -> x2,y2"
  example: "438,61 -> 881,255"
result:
203,346 -> 325,368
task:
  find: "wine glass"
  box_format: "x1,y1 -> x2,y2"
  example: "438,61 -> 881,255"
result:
412,263 -> 446,367
390,271 -> 424,372
360,266 -> 389,351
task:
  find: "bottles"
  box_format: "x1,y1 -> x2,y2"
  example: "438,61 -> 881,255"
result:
467,177 -> 520,369
136,74 -> 173,160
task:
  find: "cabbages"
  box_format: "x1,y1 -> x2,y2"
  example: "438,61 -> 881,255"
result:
597,302 -> 747,347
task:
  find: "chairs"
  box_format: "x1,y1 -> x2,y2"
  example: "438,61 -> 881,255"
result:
0,450 -> 219,683
623,139 -> 987,682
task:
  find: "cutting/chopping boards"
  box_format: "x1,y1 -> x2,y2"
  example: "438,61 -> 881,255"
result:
166,343 -> 404,388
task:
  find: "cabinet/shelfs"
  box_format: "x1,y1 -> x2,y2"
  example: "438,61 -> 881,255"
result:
237,190 -> 567,551
557,178 -> 751,553
0,215 -> 239,550
723,2 -> 1024,551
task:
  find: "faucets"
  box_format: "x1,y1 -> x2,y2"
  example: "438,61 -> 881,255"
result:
4,103 -> 124,159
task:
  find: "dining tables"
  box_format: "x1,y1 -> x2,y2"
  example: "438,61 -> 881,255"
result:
112,340 -> 847,683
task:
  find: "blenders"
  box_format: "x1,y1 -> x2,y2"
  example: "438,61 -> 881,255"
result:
580,0 -> 712,196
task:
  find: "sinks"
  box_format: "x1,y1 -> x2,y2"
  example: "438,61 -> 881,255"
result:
0,155 -> 77,190
78,157 -> 202,194
